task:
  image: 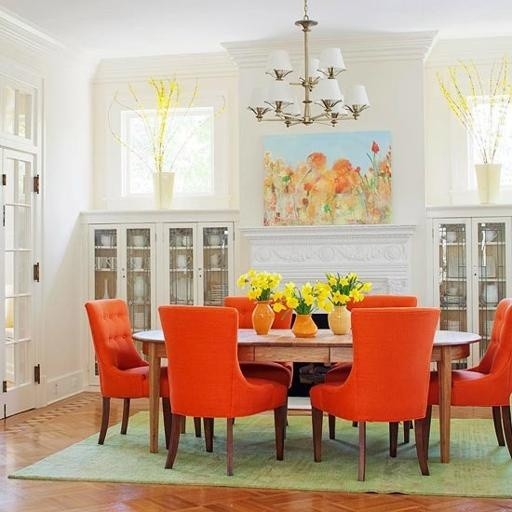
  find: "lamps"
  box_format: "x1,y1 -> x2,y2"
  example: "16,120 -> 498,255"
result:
247,0 -> 370,128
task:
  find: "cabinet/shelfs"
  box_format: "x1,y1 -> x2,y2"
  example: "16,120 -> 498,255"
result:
89,224 -> 156,385
433,216 -> 511,372
162,223 -> 233,308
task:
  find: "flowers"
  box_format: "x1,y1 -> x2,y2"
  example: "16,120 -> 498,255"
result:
284,281 -> 332,315
237,269 -> 288,313
320,268 -> 371,307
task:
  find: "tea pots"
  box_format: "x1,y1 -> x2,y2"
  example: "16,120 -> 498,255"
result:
485,228 -> 499,243
207,231 -> 221,245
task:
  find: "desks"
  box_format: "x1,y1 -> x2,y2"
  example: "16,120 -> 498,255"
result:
132,329 -> 482,465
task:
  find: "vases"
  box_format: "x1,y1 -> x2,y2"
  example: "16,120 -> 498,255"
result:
252,300 -> 275,335
328,305 -> 351,335
292,314 -> 317,338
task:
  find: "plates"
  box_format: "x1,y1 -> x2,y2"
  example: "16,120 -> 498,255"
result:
101,234 -> 116,246
208,283 -> 228,301
134,312 -> 148,329
444,320 -> 494,336
443,296 -> 463,304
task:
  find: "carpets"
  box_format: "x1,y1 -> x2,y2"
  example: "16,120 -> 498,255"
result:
7,412 -> 512,497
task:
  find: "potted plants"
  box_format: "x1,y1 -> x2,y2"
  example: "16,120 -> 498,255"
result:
106,72 -> 227,209
434,57 -> 512,205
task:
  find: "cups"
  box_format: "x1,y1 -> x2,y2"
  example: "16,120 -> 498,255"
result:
447,231 -> 457,242
446,287 -> 457,295
106,256 -> 117,270
96,257 -> 107,268
130,256 -> 144,271
176,255 -> 192,270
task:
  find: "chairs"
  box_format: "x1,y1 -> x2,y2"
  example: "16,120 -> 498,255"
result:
222,296 -> 293,425
86,298 -> 170,450
309,308 -> 440,482
158,305 -> 288,476
329,294 -> 417,445
424,298 -> 512,462
